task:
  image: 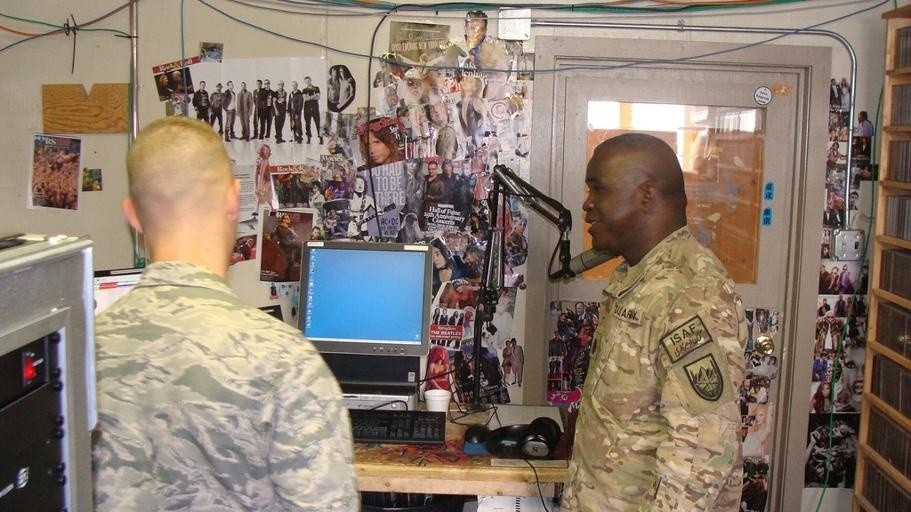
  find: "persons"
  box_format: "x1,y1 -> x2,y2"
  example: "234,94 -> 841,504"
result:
454,74 -> 489,160
266,212 -> 304,282
326,65 -> 355,112
191,76 -> 325,146
250,143 -> 279,217
394,212 -> 423,244
398,157 -> 475,227
90,112 -> 365,512
546,298 -> 602,393
420,86 -> 451,130
236,237 -> 255,260
736,307 -> 777,511
462,11 -> 488,54
554,132 -> 747,512
31,144 -> 102,212
280,141 -> 386,242
428,200 -> 530,403
157,70 -> 194,101
800,75 -> 875,488
355,116 -> 413,172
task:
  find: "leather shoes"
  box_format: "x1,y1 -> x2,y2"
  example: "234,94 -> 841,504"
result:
202,123 -> 325,147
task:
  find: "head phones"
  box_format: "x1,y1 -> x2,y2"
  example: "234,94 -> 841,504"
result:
487,417 -> 561,458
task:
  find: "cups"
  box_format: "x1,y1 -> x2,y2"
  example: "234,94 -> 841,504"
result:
424,389 -> 451,417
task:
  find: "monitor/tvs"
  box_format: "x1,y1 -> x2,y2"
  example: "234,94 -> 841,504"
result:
299,241 -> 432,356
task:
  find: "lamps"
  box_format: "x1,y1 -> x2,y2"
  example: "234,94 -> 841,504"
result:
447,161 -> 621,423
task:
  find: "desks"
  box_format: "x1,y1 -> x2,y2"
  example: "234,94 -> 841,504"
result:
350,399 -> 572,512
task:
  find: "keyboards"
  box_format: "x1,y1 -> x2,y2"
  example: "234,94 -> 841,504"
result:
346,411 -> 445,445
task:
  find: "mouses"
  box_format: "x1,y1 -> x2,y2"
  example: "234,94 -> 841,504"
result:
465,424 -> 487,444
